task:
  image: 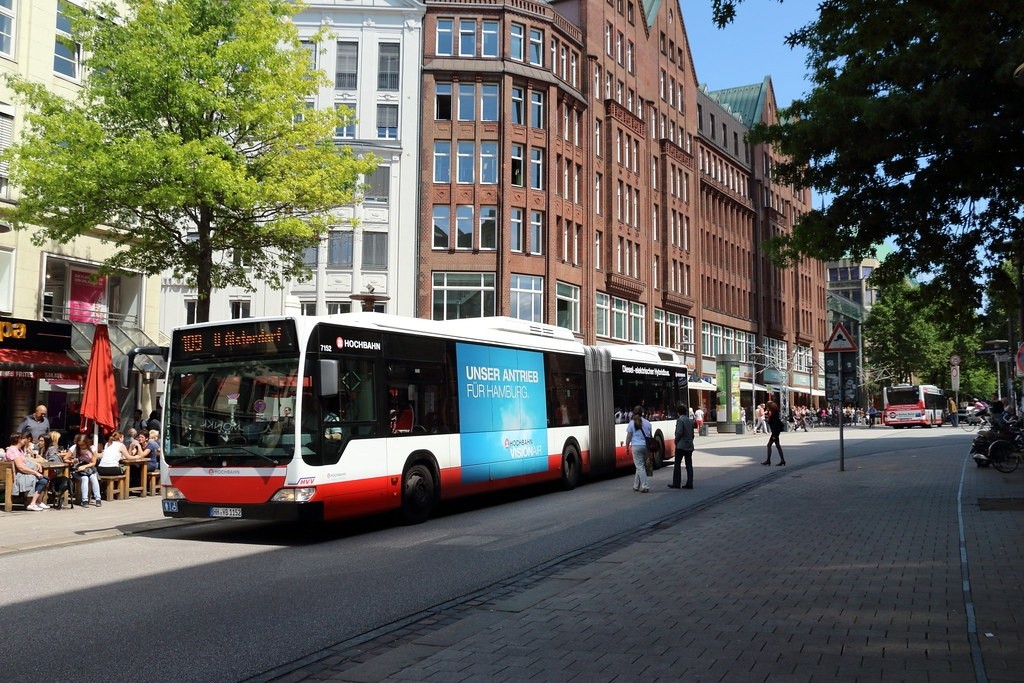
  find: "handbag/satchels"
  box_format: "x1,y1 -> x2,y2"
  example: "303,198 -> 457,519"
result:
645,458 -> 654,476
76,460 -> 93,476
641,428 -> 659,453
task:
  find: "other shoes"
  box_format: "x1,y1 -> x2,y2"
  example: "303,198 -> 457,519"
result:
96,500 -> 101,507
81,501 -> 89,508
633,488 -> 638,491
36,502 -> 50,509
26,504 -> 43,511
682,485 -> 692,489
668,484 -> 680,489
640,487 -> 649,493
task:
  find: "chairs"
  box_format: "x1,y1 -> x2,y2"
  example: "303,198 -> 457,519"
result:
396,404 -> 414,432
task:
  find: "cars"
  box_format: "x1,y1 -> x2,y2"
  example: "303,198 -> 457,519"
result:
958,410 -> 969,422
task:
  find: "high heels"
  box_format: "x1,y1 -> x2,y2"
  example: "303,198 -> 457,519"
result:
761,461 -> 771,466
775,460 -> 786,466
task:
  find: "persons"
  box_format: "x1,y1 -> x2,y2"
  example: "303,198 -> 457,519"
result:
668,405 -> 694,489
740,401 -> 865,430
301,393 -> 343,455
0,404 -> 162,511
761,403 -> 786,466
284,406 -> 292,418
689,404 -> 704,430
626,406 -> 652,493
946,396 -> 957,427
866,404 -> 876,424
970,391 -> 1014,428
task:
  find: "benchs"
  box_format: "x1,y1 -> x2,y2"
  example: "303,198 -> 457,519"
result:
0,460 -> 162,512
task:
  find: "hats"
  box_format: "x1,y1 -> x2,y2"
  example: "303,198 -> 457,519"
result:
970,397 -> 978,402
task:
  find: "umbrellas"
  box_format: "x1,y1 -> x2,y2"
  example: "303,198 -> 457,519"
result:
81,324 -> 118,455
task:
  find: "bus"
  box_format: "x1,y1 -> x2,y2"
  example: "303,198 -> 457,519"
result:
945,390 -> 972,420
882,383 -> 945,429
121,313 -> 691,524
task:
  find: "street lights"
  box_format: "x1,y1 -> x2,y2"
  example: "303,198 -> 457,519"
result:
676,335 -> 696,366
985,340 -> 1008,401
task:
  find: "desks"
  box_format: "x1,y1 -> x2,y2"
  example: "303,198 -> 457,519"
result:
98,453 -> 151,500
39,462 -> 69,505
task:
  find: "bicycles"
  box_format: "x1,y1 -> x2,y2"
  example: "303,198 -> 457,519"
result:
961,411 -> 990,433
866,413 -> 875,428
971,408 -> 1024,473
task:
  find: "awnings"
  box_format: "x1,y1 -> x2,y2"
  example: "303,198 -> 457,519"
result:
0,348 -> 88,379
